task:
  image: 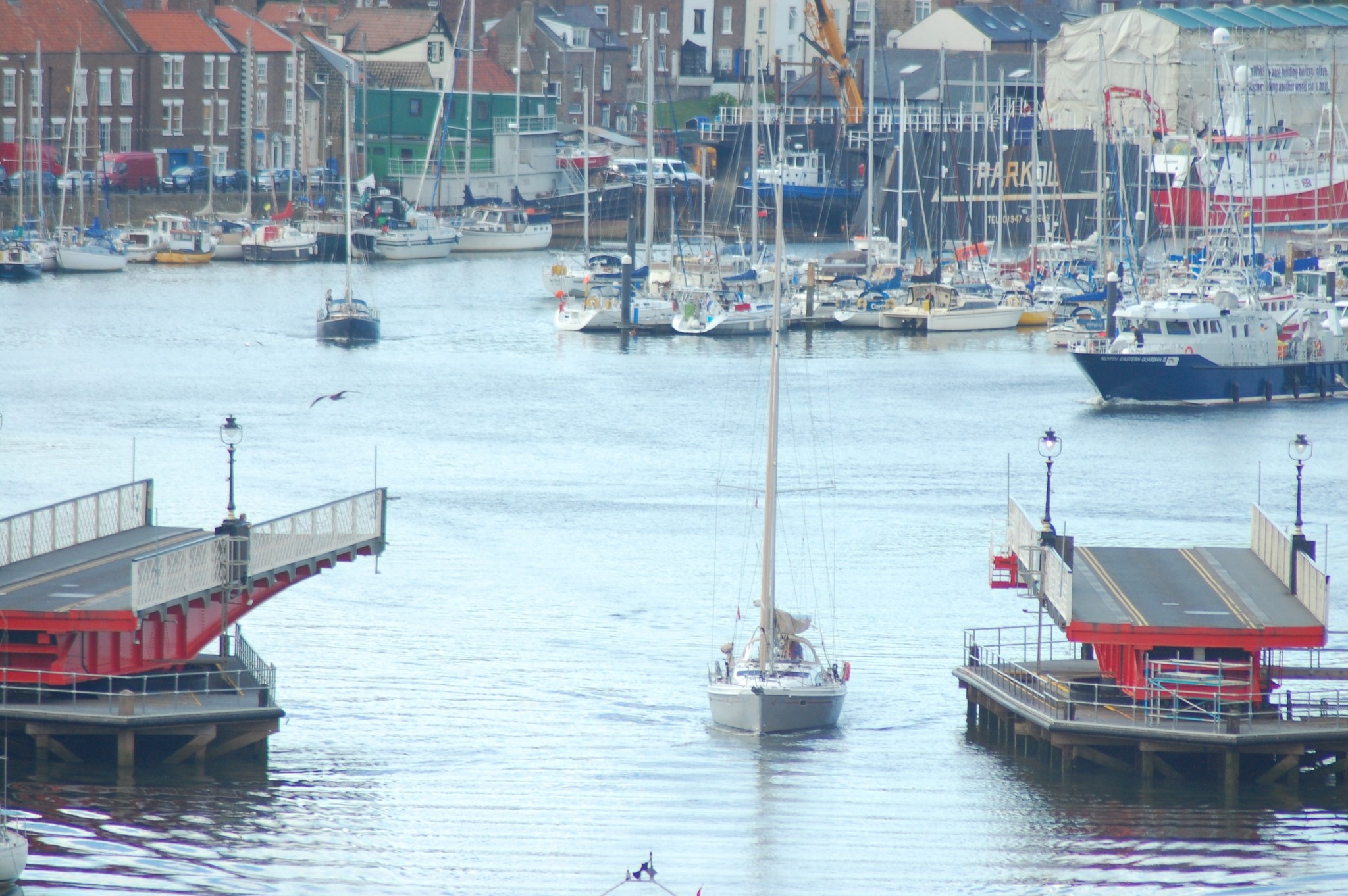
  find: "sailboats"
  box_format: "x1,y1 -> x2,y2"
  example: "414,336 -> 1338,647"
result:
0,1 -> 1348,423
700,105 -> 851,747
312,63 -> 384,347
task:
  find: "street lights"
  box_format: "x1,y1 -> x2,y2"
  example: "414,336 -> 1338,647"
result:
1288,432 -> 1314,536
1037,427 -> 1062,531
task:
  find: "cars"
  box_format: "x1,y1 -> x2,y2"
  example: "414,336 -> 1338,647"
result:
9,169 -> 57,195
251,167 -> 305,193
308,167 -> 338,190
55,168 -> 103,194
160,165 -> 217,194
214,168 -> 249,193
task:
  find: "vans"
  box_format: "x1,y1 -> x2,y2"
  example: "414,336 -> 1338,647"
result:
0,142 -> 71,177
95,150 -> 158,194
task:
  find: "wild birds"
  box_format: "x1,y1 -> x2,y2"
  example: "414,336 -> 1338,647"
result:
309,390 -> 363,409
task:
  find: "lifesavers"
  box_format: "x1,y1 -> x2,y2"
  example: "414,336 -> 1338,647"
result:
586,296 -> 599,309
1270,152 -> 1277,162
